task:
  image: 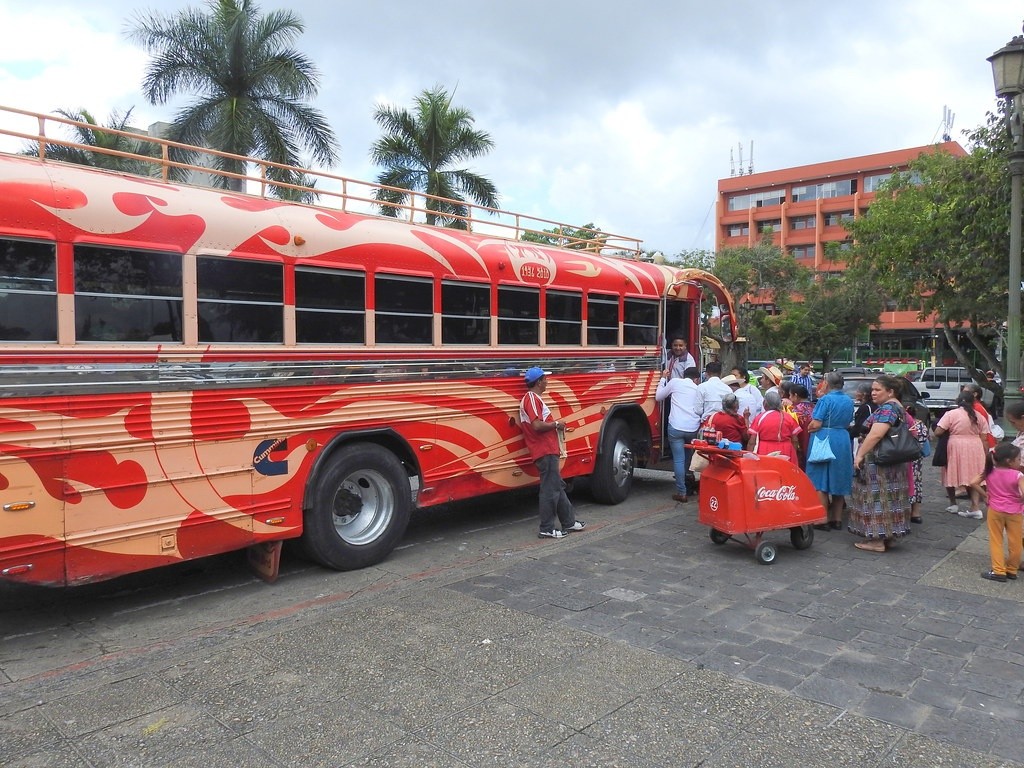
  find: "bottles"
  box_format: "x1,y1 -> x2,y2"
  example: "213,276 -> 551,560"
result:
703,427 -> 710,442
716,441 -> 741,450
708,428 -> 716,445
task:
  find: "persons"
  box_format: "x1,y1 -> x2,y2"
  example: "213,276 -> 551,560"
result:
731,366 -> 766,417
848,376 -> 910,552
847,375 -> 930,523
788,385 -> 817,457
665,335 -> 696,458
779,380 -> 793,407
935,370 -> 996,520
805,372 -> 854,531
698,362 -> 734,424
969,443 -> 1024,581
747,392 -> 803,467
791,364 -> 813,402
1005,403 -> 1024,571
700,392 -> 752,446
720,374 -> 757,427
655,367 -> 704,502
519,366 -> 588,539
759,365 -> 783,395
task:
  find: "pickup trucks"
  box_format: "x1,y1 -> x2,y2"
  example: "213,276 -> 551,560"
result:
912,367 -> 1002,420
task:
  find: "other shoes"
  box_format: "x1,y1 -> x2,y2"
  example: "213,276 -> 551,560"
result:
1006,572 -> 1017,579
911,517 -> 922,523
855,542 -> 885,552
829,520 -> 841,529
946,505 -> 958,513
981,570 -> 1006,582
672,494 -> 688,502
814,523 -> 830,531
958,510 -> 983,519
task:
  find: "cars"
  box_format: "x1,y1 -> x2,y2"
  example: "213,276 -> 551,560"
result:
841,372 -> 891,425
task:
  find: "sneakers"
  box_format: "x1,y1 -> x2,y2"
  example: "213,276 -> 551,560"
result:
539,529 -> 568,538
562,521 -> 587,532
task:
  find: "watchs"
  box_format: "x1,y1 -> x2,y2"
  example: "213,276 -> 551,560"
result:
555,421 -> 559,427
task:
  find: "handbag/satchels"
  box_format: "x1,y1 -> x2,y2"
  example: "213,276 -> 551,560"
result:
689,449 -> 709,473
981,405 -> 1004,441
807,436 -> 836,463
873,402 -> 922,466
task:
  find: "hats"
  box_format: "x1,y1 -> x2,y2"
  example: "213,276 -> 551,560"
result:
759,366 -> 784,388
721,375 -> 746,385
525,367 -> 552,384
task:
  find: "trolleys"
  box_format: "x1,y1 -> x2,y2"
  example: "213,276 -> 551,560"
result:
684,428 -> 828,566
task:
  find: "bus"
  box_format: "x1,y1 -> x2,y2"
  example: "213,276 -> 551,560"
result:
0,102 -> 742,593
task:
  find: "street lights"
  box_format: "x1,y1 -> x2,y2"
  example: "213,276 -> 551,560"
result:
984,34 -> 1024,441
